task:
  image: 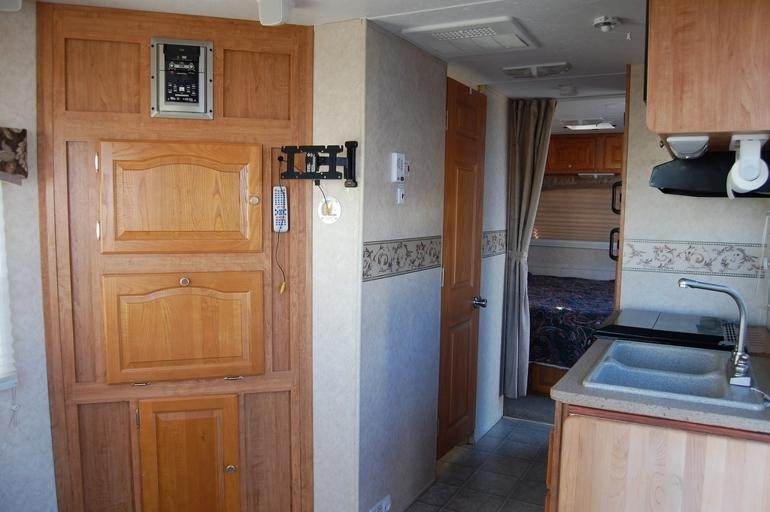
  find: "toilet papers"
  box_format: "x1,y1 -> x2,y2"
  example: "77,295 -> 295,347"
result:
725,158 -> 769,199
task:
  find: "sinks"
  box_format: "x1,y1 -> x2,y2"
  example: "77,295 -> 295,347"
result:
583,360 -> 730,405
603,340 -> 726,376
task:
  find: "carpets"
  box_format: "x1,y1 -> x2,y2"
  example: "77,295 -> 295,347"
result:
502,388 -> 561,427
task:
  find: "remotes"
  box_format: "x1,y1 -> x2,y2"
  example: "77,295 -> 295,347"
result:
272,186 -> 289,233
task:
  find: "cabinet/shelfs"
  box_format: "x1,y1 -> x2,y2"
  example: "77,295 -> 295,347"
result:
643,0 -> 770,160
542,397 -> 770,511
593,132 -> 624,174
545,133 -> 593,174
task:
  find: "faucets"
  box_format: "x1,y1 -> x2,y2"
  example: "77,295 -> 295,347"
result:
677,276 -> 750,377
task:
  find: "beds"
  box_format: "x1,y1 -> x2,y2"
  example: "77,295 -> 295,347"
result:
527,268 -> 617,397
